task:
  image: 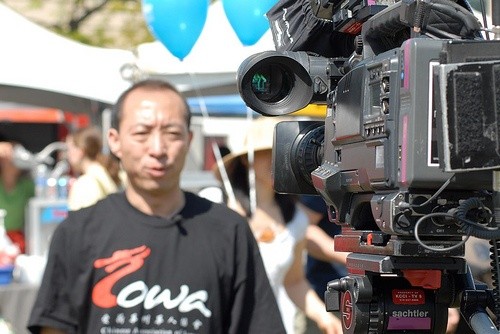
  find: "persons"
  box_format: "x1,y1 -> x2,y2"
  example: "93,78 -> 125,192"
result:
0,78 -> 350,334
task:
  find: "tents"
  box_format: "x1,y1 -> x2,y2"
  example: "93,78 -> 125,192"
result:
0,0 -> 301,164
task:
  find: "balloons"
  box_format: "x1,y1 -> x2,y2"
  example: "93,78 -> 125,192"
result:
222,0 -> 285,48
142,1 -> 211,61
